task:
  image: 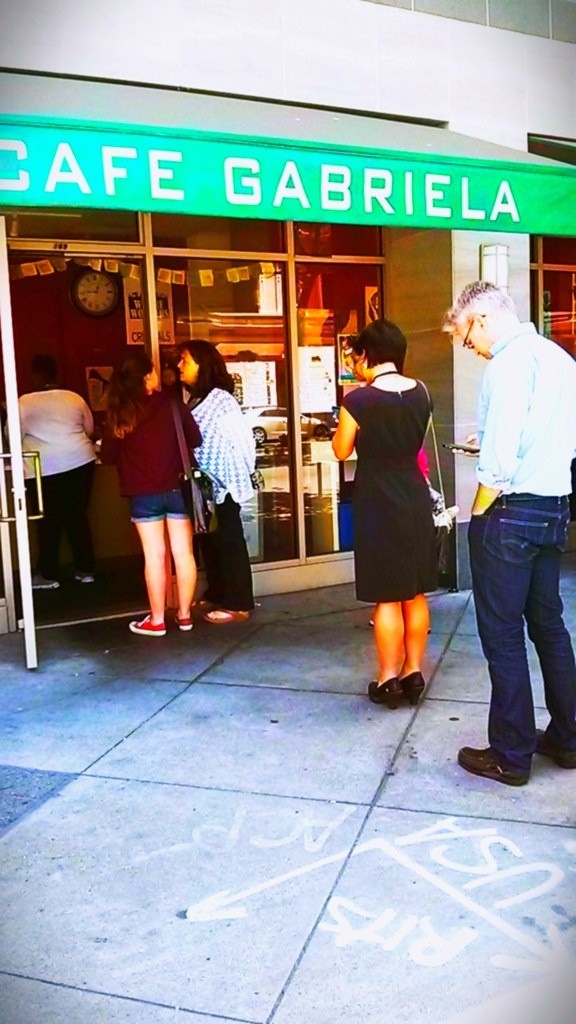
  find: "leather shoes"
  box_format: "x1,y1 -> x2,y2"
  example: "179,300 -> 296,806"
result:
458,747 -> 529,786
535,728 -> 576,769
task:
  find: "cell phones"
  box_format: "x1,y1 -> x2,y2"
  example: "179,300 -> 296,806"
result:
442,443 -> 480,454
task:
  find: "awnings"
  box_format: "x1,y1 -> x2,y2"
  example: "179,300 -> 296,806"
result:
0,68 -> 576,236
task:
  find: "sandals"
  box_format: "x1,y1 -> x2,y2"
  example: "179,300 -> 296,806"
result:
203,608 -> 250,624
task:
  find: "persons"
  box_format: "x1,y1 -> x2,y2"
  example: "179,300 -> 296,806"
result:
4,353 -> 97,589
331,319 -> 445,710
341,337 -> 355,373
439,279 -> 576,786
369,446 -> 432,635
101,354 -> 202,637
177,340 -> 254,623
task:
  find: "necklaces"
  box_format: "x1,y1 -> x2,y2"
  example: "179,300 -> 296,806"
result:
373,371 -> 399,380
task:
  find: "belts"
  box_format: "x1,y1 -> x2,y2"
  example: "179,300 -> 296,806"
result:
505,491 -> 542,503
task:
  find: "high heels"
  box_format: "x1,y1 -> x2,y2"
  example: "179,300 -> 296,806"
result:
401,671 -> 426,706
368,677 -> 401,710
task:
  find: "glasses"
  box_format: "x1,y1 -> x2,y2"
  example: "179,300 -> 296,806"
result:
463,313 -> 487,350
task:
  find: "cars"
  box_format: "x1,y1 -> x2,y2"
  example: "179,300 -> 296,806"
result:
246,407 -> 331,444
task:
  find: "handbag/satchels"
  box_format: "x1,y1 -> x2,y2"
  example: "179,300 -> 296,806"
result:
427,486 -> 443,515
179,467 -> 215,533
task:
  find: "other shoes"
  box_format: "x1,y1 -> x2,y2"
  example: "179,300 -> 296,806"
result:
31,574 -> 60,589
74,569 -> 94,583
128,614 -> 166,637
175,613 -> 193,632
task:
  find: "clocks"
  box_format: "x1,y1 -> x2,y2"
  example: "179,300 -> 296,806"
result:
68,267 -> 120,321
